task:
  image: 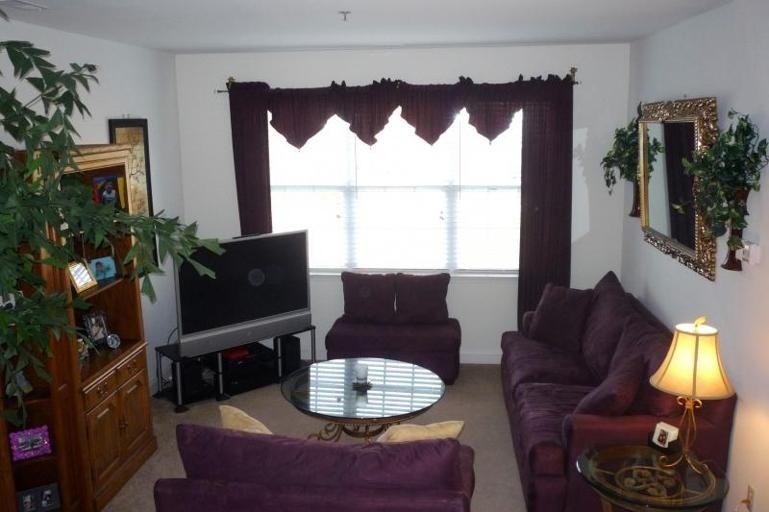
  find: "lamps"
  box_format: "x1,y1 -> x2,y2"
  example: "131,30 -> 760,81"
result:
649,321 -> 736,475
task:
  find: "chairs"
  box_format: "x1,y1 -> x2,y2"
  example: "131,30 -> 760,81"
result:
153,424 -> 474,512
324,271 -> 462,384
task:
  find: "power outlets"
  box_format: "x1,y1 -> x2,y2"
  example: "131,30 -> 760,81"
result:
746,485 -> 755,512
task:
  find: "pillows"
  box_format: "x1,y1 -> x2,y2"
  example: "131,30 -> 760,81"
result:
376,420 -> 465,444
175,424 -> 459,490
217,405 -> 272,434
341,272 -> 397,323
396,273 -> 450,324
529,271 -> 686,418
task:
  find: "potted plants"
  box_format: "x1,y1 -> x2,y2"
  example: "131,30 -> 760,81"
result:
600,116 -> 640,218
672,107 -> 769,272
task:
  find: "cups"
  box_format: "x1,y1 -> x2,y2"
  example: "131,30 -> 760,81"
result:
355,364 -> 368,384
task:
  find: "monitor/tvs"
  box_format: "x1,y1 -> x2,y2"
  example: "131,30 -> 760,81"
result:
173,229 -> 312,358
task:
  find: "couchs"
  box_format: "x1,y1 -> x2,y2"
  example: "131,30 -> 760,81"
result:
500,292 -> 738,512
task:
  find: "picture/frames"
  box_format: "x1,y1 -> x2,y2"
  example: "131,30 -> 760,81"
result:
108,118 -> 159,279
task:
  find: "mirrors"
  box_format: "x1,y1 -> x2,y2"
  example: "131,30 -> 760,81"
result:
637,96 -> 716,281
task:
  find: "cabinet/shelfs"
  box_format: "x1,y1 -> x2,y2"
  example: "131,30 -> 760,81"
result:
152,325 -> 316,413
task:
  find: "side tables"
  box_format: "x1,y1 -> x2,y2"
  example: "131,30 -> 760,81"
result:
575,440 -> 730,512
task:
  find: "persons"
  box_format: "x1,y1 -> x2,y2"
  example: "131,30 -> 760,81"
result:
102,180 -> 119,205
